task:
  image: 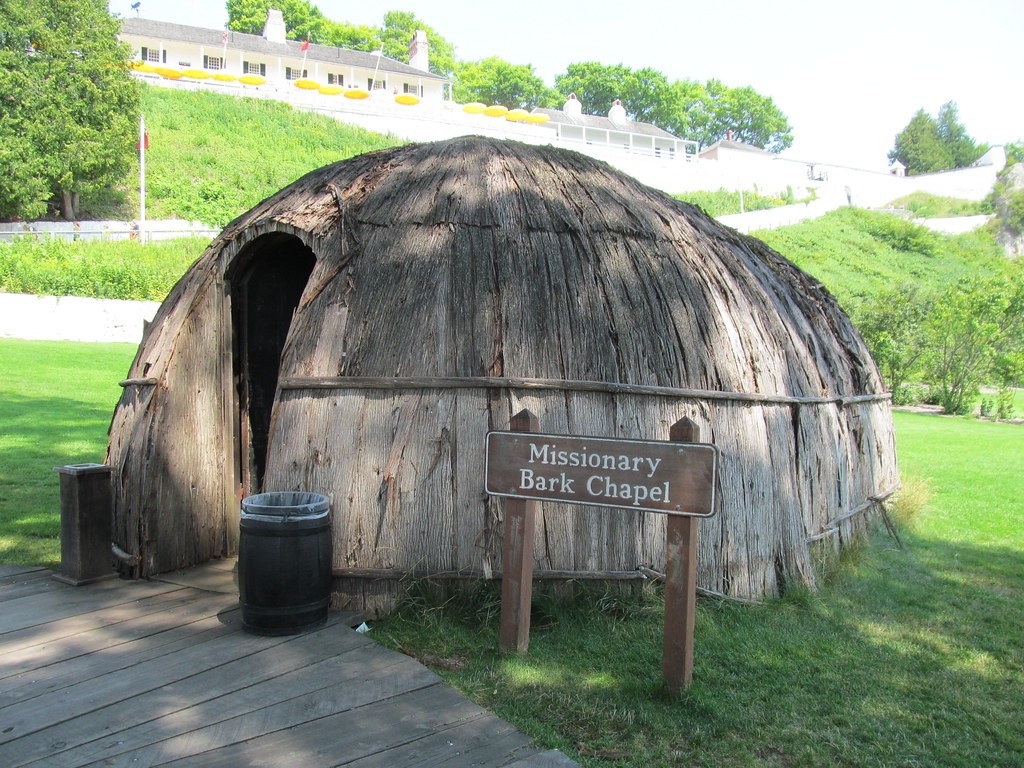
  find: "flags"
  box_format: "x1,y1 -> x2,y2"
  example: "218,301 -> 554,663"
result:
300,38 -> 309,52
370,49 -> 381,56
222,28 -> 228,45
136,120 -> 149,150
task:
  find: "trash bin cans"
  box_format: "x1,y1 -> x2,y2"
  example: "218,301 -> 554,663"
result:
236,492 -> 334,637
